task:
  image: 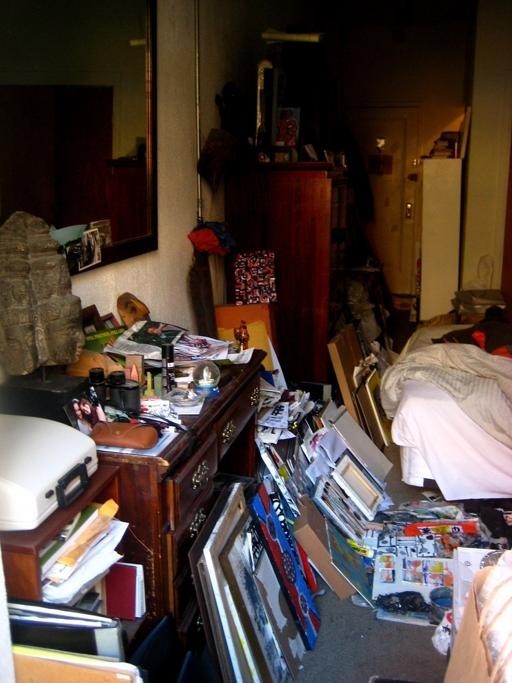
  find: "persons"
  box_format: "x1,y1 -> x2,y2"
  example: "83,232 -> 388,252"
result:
72,397 -> 106,435
145,325 -> 181,346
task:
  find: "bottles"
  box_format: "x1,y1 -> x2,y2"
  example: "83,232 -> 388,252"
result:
161,344 -> 177,397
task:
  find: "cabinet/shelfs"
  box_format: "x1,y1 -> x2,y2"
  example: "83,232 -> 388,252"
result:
1,351 -> 268,682
0,85 -> 148,242
225,159 -> 360,386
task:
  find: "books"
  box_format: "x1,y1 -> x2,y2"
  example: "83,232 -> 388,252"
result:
37,502 -> 148,622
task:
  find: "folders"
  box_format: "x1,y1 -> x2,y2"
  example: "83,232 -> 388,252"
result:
5,595 -> 143,682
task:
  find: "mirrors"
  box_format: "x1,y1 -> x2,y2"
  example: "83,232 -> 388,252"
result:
1,2 -> 157,276
